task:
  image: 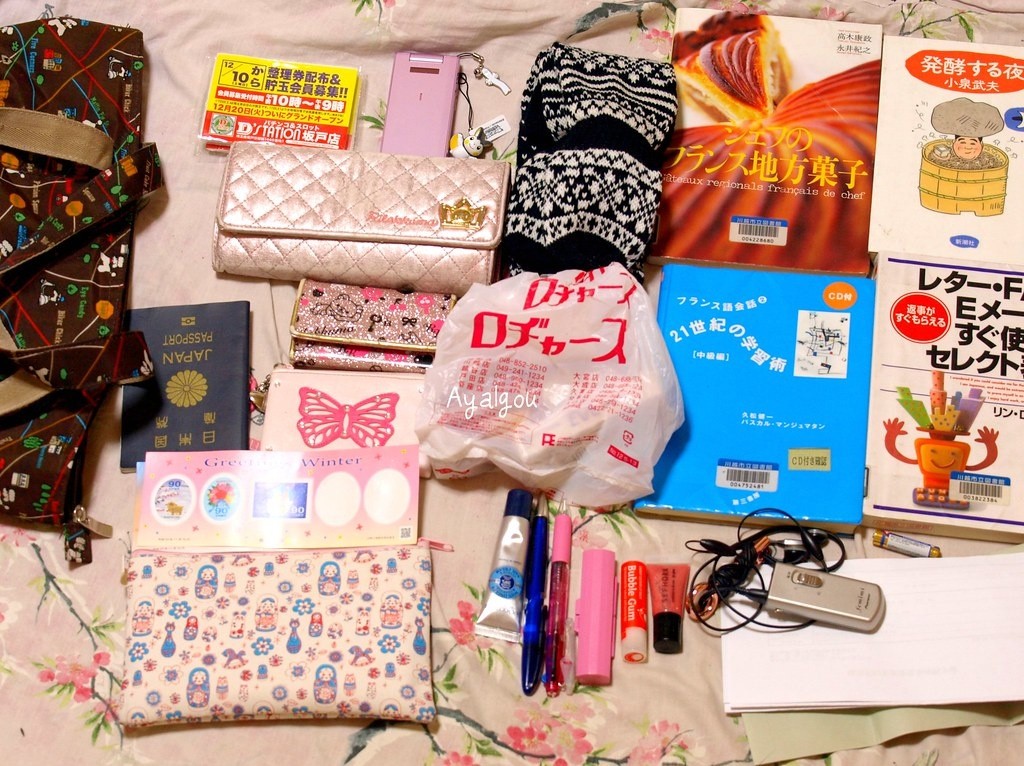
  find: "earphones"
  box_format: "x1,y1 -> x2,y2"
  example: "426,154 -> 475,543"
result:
801,526 -> 829,546
780,546 -> 807,565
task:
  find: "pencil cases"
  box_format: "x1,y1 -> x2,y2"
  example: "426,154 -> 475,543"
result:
115,537 -> 454,726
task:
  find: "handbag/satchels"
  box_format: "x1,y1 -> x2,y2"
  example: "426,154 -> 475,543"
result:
0,16 -> 165,565
410,261 -> 684,506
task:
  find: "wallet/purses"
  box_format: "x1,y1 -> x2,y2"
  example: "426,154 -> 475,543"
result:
213,141 -> 510,294
290,276 -> 456,372
119,541 -> 437,733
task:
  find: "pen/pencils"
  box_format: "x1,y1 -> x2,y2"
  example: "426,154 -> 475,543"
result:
520,489 -> 551,699
543,490 -> 573,696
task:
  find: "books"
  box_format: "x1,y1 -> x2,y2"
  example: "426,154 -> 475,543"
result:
864,252 -> 1024,544
634,263 -> 876,538
119,300 -> 249,473
648,6 -> 882,278
867,35 -> 1024,264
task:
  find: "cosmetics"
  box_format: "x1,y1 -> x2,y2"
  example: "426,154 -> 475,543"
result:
622,561 -> 646,664
647,563 -> 690,654
474,488 -> 533,643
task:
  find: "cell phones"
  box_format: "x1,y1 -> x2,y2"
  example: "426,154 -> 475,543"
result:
380,52 -> 462,158
